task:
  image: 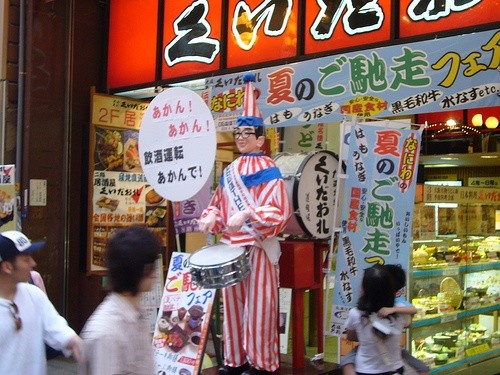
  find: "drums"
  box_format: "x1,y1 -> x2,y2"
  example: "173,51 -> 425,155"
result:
274,151 -> 348,239
189,244 -> 249,287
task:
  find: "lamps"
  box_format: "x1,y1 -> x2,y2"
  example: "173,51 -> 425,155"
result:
472,114 -> 483,127
485,116 -> 499,130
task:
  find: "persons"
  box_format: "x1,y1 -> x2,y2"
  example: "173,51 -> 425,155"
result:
338,263 -> 430,375
0,231 -> 83,375
28,269 -> 48,297
76,223 -> 164,375
196,116 -> 293,375
342,266 -> 406,375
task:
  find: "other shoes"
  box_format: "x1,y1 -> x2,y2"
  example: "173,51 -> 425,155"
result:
219,362 -> 275,375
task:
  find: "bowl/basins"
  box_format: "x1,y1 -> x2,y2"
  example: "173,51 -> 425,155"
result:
411,237 -> 500,366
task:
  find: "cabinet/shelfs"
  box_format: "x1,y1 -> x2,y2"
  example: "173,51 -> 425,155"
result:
403,231 -> 500,375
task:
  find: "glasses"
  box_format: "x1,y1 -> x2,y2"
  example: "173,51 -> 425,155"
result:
7,301 -> 22,330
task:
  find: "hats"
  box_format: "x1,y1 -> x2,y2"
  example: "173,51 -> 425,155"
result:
0,230 -> 45,264
237,72 -> 264,127
